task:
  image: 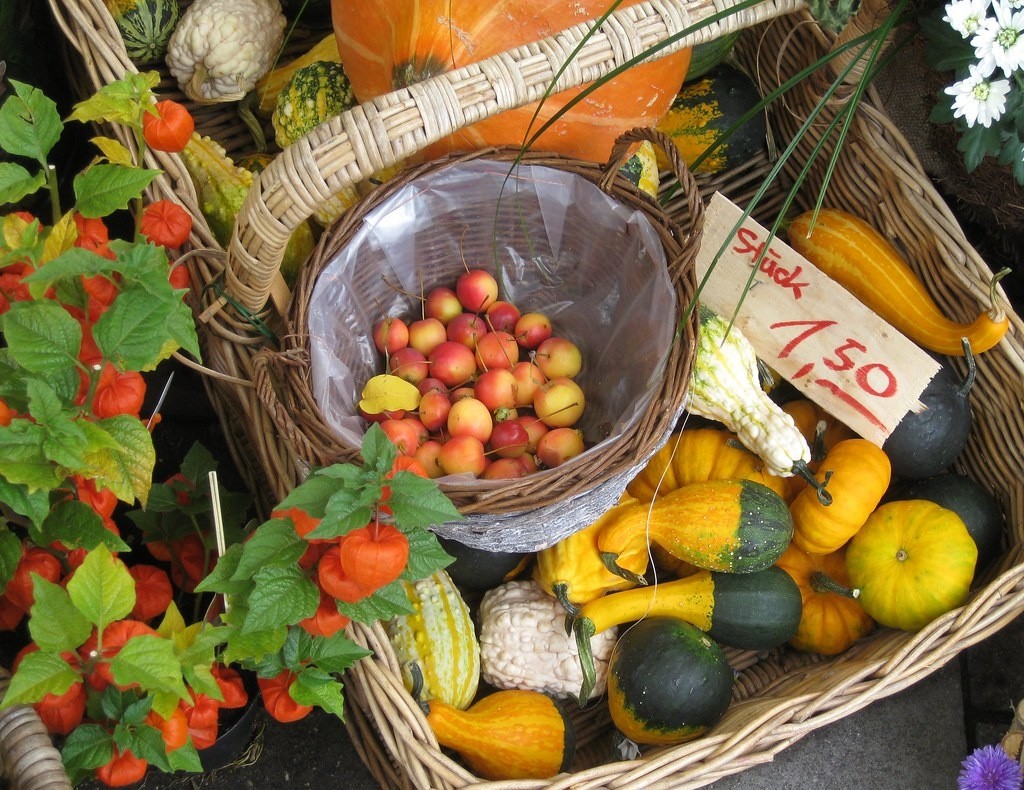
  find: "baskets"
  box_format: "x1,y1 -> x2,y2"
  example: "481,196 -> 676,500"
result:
45,0 -> 1024,790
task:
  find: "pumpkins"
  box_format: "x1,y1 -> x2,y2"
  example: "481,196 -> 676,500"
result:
383,207 -> 1009,777
110,0 -> 766,274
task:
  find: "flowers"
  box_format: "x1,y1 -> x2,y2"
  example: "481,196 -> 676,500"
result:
957,744 -> 1022,790
941,0 -> 1024,187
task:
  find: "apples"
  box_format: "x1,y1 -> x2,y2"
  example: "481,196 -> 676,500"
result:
357,270 -> 583,477
